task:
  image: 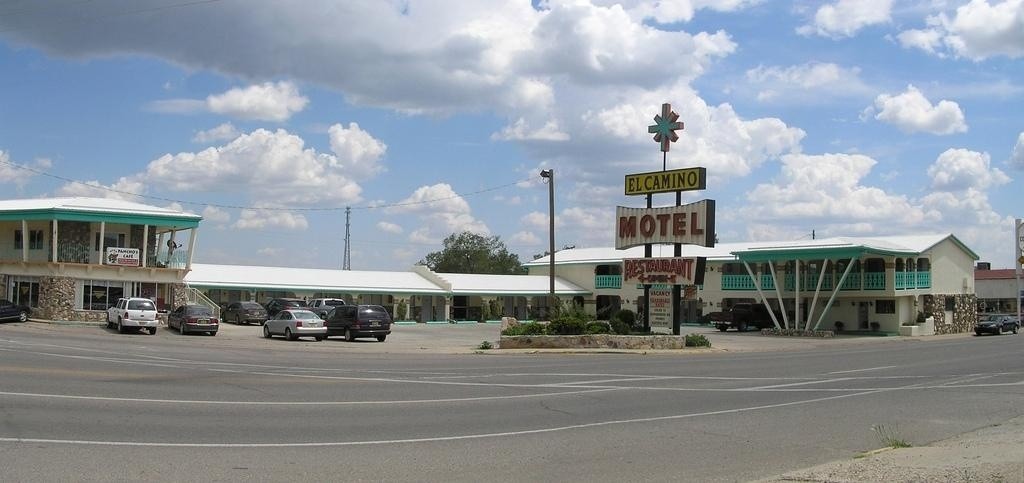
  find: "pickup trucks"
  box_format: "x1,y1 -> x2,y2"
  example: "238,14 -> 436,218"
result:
708,301 -> 770,331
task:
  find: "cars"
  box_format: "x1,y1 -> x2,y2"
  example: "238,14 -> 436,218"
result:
0,298 -> 31,324
220,300 -> 268,325
974,312 -> 1018,334
262,309 -> 328,341
167,303 -> 219,337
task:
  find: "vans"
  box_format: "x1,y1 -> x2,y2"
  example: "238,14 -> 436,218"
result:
320,303 -> 391,342
308,298 -> 346,317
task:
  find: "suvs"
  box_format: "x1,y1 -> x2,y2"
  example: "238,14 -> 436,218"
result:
105,296 -> 161,335
258,296 -> 308,324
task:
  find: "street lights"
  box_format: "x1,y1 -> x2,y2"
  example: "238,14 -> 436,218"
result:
538,168 -> 554,294
